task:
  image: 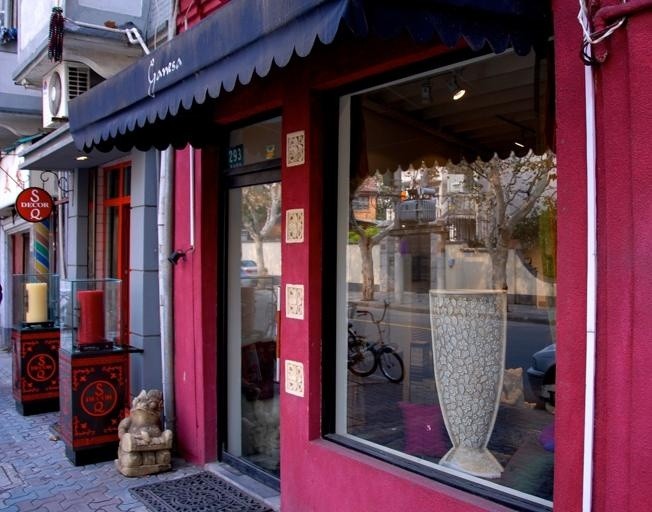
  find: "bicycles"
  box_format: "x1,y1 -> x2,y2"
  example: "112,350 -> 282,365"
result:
347,298 -> 406,385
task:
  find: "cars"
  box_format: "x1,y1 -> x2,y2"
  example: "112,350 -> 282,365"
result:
526,340 -> 557,407
241,255 -> 271,279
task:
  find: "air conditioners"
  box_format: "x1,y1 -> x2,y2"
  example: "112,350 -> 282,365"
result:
40,58 -> 92,132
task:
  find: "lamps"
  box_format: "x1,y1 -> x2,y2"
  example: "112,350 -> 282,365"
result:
442,71 -> 474,105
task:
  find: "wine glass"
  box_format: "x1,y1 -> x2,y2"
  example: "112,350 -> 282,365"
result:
427,288 -> 507,479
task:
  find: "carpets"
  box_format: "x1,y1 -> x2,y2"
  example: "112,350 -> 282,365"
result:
125,469 -> 281,512
